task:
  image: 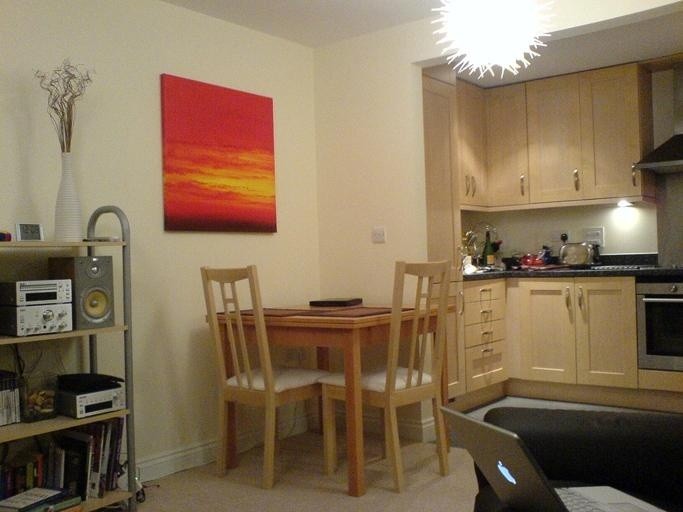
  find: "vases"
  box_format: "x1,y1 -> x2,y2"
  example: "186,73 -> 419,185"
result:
53,152 -> 82,244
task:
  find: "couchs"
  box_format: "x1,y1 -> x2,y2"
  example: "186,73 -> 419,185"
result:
474,408 -> 683,512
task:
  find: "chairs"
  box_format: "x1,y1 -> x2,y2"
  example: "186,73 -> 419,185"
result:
317,260 -> 453,495
199,264 -> 332,486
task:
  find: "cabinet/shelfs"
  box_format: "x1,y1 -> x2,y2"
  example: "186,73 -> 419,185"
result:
524,61 -> 657,209
429,277 -> 508,414
485,83 -> 530,213
457,76 -> 487,214
423,73 -> 463,283
0,205 -> 137,512
507,277 -> 638,410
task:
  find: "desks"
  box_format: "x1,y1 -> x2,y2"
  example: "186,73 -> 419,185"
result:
205,305 -> 458,496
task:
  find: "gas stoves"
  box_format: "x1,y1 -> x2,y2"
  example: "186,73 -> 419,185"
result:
636,264 -> 683,294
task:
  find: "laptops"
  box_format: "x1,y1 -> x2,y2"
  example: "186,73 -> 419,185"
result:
441,406 -> 670,512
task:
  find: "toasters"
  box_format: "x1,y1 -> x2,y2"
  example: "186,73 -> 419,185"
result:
560,242 -> 601,269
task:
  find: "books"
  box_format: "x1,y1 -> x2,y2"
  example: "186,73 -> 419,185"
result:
0,370 -> 23,428
0,414 -> 126,512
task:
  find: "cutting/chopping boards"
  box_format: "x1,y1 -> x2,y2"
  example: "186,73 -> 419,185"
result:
519,264 -> 559,270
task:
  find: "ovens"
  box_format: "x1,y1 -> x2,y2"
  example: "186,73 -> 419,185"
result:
637,295 -> 683,372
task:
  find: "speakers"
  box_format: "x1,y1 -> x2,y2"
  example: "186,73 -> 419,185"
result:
46,256 -> 114,330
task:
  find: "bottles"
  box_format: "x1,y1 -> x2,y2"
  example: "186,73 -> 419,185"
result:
482,225 -> 496,272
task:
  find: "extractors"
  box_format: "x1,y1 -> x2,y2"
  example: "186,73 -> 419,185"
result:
634,133 -> 683,175
